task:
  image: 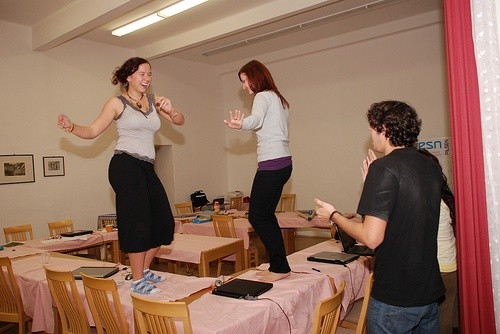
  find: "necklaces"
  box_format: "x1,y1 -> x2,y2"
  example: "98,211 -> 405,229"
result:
127,92 -> 146,108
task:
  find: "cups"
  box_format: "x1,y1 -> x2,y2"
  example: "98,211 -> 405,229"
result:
106,225 -> 113,232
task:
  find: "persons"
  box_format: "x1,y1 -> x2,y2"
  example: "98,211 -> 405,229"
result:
314,101 -> 446,334
224,59 -> 292,283
59,57 -> 184,294
361,136 -> 459,334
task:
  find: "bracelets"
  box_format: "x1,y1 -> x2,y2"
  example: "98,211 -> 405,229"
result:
329,211 -> 341,225
65,122 -> 74,133
172,112 -> 177,118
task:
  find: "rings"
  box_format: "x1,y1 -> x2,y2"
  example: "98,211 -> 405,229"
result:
234,119 -> 237,120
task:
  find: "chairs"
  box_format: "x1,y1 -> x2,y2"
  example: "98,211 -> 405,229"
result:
310,280 -> 347,334
129,293 -> 193,334
211,214 -> 259,277
47,219 -> 78,255
43,265 -> 98,334
279,194 -> 299,241
79,271 -> 129,334
0,257 -> 32,334
230,196 -> 244,211
339,271 -> 374,334
174,202 -> 193,215
3,224 -> 33,243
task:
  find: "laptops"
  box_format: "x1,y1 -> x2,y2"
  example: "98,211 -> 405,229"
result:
72,267 -> 120,279
60,230 -> 93,237
335,224 -> 376,256
307,251 -> 359,264
212,278 -> 273,299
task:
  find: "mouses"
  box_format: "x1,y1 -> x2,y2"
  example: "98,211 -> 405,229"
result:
125,274 -> 133,280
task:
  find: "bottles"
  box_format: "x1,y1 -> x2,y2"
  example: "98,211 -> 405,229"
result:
214,201 -> 220,213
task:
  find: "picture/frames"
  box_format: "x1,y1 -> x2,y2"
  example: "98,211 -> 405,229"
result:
0,154 -> 35,185
43,156 -> 66,177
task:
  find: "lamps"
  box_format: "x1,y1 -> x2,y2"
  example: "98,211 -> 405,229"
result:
111,0 -> 405,57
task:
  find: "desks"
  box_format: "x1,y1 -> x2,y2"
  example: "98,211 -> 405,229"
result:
0,211 -> 374,334
